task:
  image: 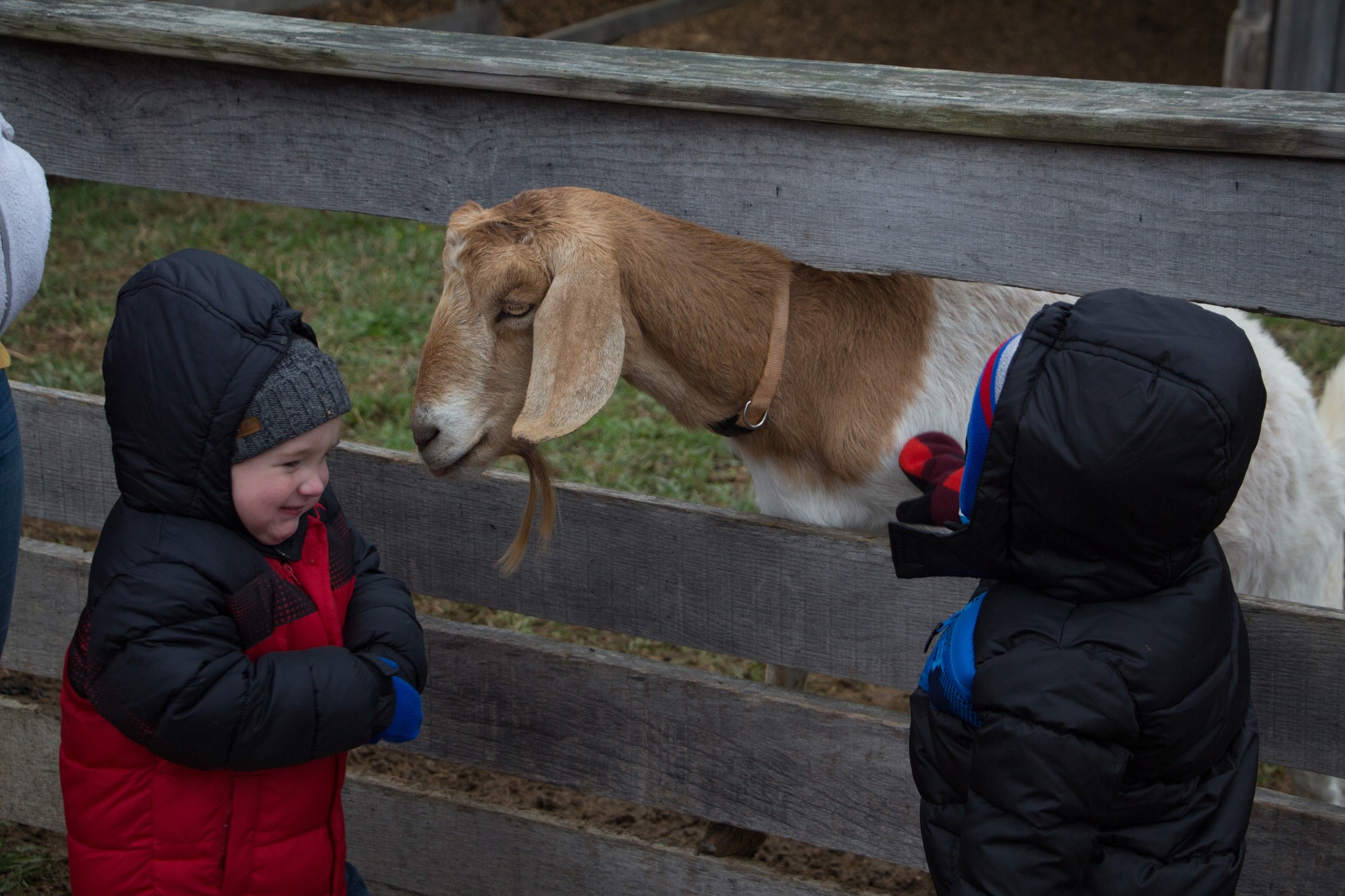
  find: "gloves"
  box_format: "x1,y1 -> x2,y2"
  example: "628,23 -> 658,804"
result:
379,656 -> 422,744
896,430 -> 966,524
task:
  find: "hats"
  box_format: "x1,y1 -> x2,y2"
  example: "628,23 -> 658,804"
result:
232,332 -> 355,467
959,334 -> 1023,524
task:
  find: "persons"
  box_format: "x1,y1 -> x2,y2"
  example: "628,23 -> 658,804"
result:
0,118 -> 52,653
888,287 -> 1267,896
58,247 -> 427,896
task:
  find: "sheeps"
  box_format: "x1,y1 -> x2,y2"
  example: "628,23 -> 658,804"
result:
406,178 -> 1345,866
